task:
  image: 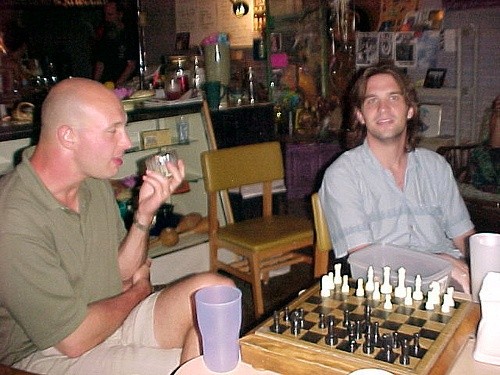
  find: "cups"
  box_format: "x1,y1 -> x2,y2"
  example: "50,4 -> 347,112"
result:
469,232 -> 500,304
202,81 -> 225,111
176,115 -> 192,144
204,41 -> 231,87
194,285 -> 242,373
253,38 -> 266,59
146,149 -> 177,179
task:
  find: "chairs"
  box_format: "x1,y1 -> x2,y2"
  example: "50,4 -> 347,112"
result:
200,142 -> 333,323
435,95 -> 500,234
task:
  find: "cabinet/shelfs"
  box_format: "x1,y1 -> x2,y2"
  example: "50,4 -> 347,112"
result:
0,97 -> 245,287
353,23 -> 479,169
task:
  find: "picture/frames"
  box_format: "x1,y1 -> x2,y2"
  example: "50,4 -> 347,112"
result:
422,66 -> 448,90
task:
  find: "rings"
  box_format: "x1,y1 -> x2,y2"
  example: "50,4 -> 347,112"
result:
460,272 -> 466,277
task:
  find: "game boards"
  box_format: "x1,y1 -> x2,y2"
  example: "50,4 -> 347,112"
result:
255,276 -> 473,375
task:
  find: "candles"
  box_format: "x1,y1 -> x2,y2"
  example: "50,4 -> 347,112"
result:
139,10 -> 147,26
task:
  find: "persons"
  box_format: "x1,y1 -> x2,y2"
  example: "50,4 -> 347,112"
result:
359,41 -> 373,64
0,26 -> 28,66
94,2 -> 138,84
381,34 -> 391,57
488,97 -> 500,177
319,64 -> 475,294
0,77 -> 235,375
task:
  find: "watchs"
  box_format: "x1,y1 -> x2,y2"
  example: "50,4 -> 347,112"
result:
133,212 -> 156,230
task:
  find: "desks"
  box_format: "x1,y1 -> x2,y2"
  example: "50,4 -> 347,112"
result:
172,276 -> 500,375
239,126 -> 342,219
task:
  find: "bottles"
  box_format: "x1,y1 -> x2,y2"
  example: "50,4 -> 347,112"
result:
163,55 -> 206,99
